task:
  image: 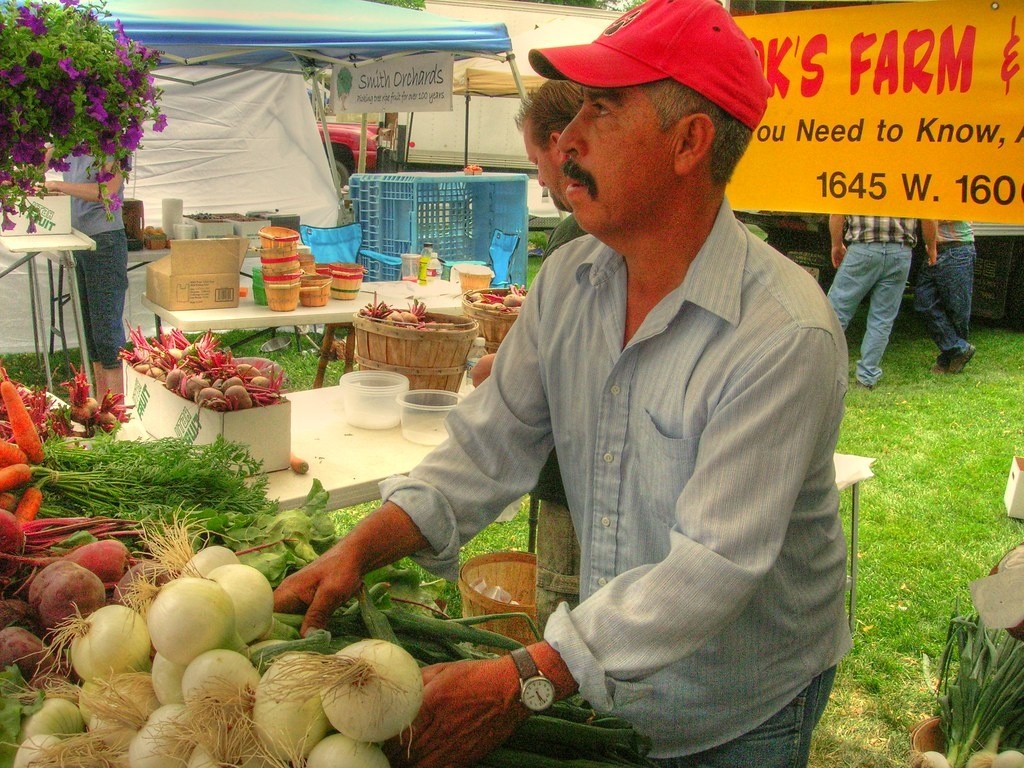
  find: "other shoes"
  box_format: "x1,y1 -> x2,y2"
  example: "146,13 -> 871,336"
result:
854,379 -> 874,391
930,364 -> 945,374
948,345 -> 976,375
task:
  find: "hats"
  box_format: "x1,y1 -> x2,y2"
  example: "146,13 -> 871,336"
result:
527,0 -> 773,134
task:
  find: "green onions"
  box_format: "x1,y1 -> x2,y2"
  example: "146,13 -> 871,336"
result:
933,597 -> 1024,768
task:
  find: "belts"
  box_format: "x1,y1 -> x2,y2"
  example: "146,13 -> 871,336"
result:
936,241 -> 970,250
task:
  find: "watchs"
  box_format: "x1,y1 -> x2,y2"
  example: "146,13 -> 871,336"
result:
511,648 -> 555,711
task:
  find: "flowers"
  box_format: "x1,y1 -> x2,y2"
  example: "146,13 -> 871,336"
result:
0,0 -> 168,231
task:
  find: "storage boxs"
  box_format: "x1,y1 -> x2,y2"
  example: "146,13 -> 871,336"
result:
348,172 -> 530,288
146,234 -> 250,311
122,360 -> 291,479
461,288 -> 528,355
351,312 -> 479,412
1003,456 -> 1024,520
222,215 -> 271,245
249,214 -> 301,232
183,215 -> 234,239
458,553 -> 536,657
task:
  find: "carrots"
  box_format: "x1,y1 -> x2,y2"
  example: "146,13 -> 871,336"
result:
0,382 -> 44,532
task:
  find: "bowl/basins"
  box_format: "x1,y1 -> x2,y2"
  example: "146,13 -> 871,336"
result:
172,223 -> 194,240
161,197 -> 184,236
396,388 -> 463,446
339,370 -> 410,430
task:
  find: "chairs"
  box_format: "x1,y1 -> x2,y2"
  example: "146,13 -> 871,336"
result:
833,453 -> 875,632
484,228 -> 521,287
300,222 -> 403,282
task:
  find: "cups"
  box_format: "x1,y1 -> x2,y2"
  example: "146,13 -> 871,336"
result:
400,253 -> 420,283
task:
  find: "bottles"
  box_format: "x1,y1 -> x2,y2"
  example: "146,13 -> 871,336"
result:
426,252 -> 443,291
464,336 -> 489,401
416,243 -> 433,286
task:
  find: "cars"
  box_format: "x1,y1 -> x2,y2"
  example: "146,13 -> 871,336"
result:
317,119 -> 378,186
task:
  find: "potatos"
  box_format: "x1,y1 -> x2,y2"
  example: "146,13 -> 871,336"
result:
0,540 -> 166,671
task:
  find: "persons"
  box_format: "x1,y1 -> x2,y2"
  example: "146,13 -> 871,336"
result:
34,131 -> 128,407
472,76 -> 584,640
914,219 -> 976,374
272,0 -> 853,768
824,213 -> 937,388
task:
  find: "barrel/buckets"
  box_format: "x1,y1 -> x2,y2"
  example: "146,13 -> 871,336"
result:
259,336 -> 292,354
909,715 -> 945,763
457,551 -> 538,654
329,261 -> 368,301
295,240 -> 330,278
460,287 -> 529,355
120,197 -> 144,239
298,274 -> 333,307
351,307 -> 480,415
257,225 -> 305,312
988,539 -> 1024,643
453,264 -> 493,300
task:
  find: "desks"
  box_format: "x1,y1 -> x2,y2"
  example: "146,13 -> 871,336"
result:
1,227 -> 96,399
77,385 -> 539,555
48,242 -> 311,377
143,279 -> 464,390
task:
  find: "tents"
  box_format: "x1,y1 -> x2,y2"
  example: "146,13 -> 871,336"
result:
0,0 -> 566,356
